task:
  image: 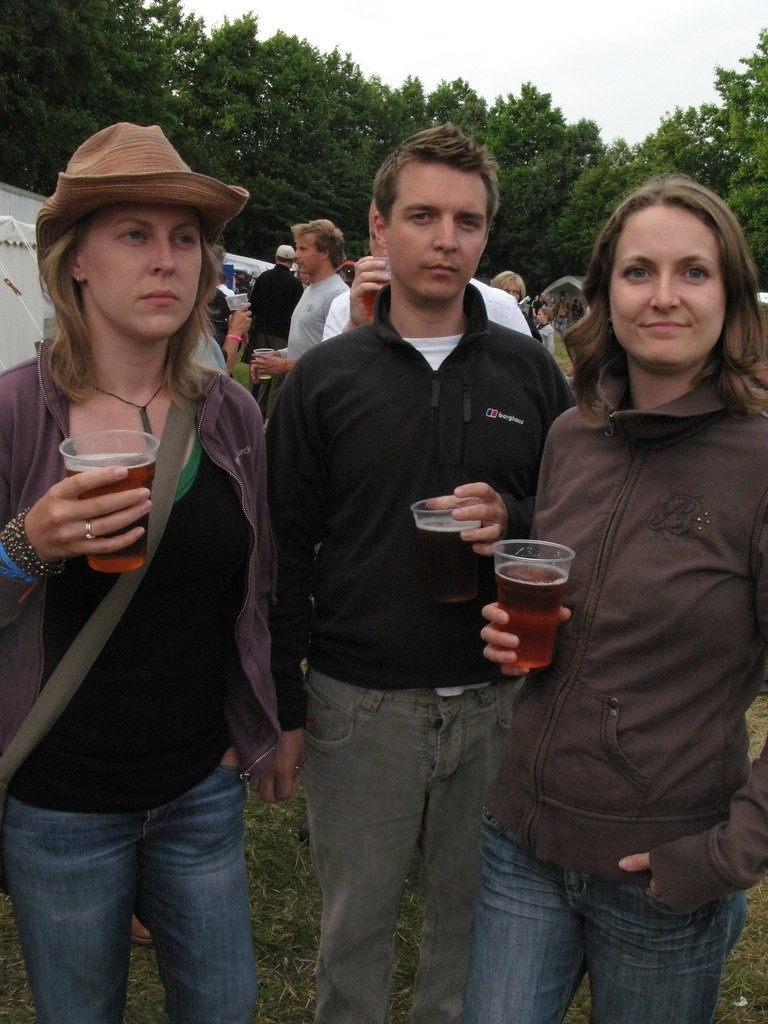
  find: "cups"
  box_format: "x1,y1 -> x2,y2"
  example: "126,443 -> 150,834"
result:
59,429 -> 159,573
363,257 -> 391,322
254,348 -> 273,379
225,293 -> 248,316
492,539 -> 575,668
411,497 -> 481,602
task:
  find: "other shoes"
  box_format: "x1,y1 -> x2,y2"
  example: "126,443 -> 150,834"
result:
300,818 -> 310,845
131,913 -> 153,944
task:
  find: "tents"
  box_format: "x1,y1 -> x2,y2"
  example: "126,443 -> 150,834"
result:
1,216 -> 48,379
222,245 -> 300,285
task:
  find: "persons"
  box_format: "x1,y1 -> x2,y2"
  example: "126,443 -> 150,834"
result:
491,267 -> 586,392
0,124 -> 274,1024
259,120 -> 571,1024
129,198 -> 395,949
483,178 -> 768,1024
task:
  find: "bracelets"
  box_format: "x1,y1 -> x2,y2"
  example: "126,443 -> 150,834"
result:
0,505 -> 66,583
226,335 -> 242,342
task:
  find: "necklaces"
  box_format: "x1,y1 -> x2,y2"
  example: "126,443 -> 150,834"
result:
85,379 -> 164,448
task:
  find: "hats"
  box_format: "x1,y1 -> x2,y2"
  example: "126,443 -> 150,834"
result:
339,260 -> 356,270
35,123 -> 249,283
276,245 -> 295,258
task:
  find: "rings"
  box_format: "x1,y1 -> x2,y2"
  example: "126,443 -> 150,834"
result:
84,520 -> 95,540
492,523 -> 501,534
